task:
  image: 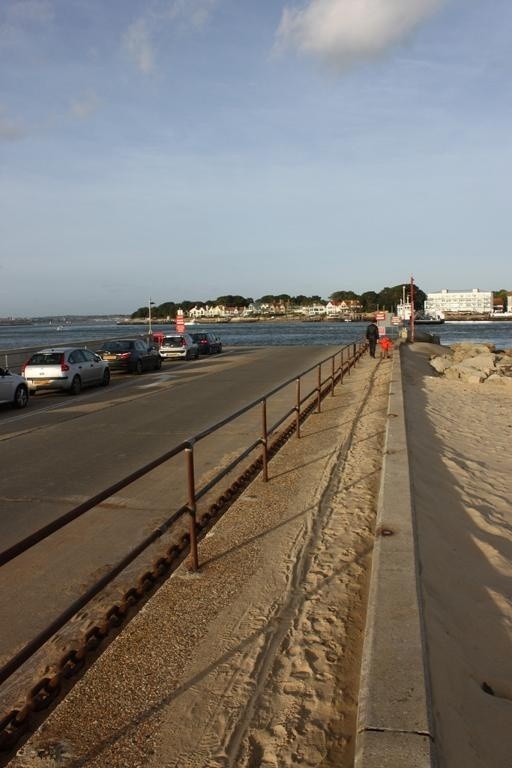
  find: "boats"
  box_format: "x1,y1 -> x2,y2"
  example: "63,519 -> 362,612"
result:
408,311 -> 445,324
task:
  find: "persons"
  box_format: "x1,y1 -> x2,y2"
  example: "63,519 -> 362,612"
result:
366,319 -> 379,358
378,335 -> 392,359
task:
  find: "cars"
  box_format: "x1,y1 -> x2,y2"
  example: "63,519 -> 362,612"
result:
98,338 -> 163,374
19,346 -> 111,396
188,330 -> 224,355
158,332 -> 200,362
0,365 -> 31,410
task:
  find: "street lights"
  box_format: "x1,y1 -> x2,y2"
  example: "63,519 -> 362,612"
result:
147,296 -> 156,334
